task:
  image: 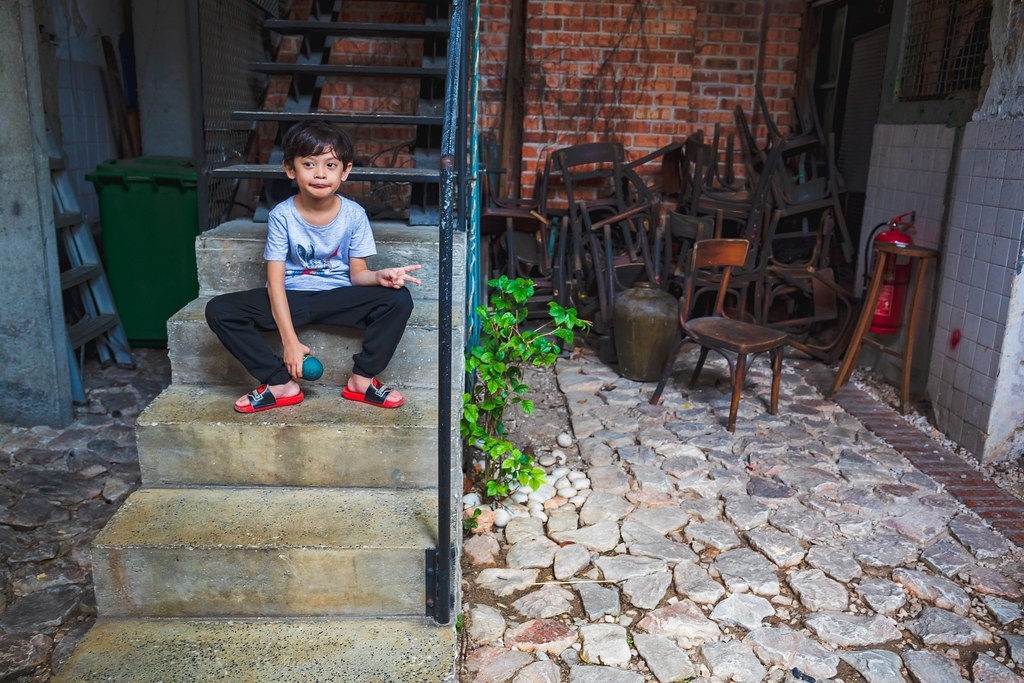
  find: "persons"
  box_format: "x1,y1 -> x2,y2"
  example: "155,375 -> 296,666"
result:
204,117 -> 422,413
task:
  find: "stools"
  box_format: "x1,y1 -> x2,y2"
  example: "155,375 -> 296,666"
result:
832,239 -> 939,417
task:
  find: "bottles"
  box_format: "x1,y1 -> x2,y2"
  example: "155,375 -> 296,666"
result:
612,282 -> 681,384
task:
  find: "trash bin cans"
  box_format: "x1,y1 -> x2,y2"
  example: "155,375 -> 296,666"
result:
83,155 -> 200,348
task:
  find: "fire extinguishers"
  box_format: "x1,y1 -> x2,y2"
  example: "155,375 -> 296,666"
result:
860,210 -> 917,335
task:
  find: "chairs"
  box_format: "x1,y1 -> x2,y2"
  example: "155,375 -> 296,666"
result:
648,238 -> 790,434
480,63 -> 863,366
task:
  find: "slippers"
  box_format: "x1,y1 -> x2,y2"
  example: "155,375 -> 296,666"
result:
235,384 -> 304,413
341,377 -> 404,409
